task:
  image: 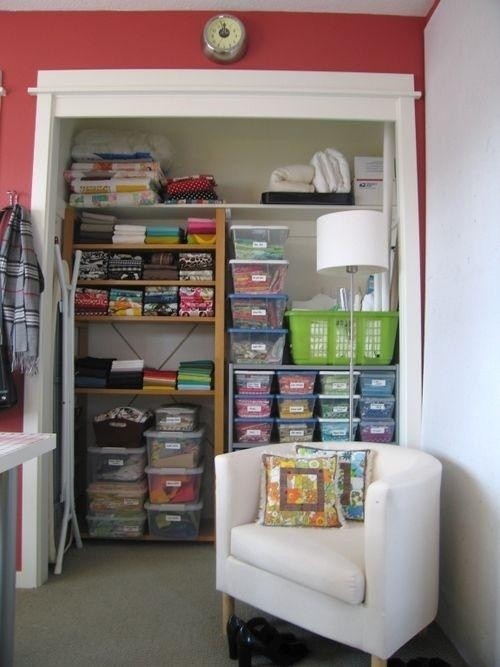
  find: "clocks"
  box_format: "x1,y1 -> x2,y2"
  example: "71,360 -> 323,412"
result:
201,12 -> 249,65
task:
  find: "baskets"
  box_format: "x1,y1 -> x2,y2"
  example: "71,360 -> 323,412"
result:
284,309 -> 399,366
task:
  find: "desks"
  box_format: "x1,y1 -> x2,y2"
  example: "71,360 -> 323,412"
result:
0,431 -> 57,667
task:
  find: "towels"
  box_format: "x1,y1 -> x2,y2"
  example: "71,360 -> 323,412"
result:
269,147 -> 353,193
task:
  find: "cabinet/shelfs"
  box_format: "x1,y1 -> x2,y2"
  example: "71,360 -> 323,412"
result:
60,207 -> 226,544
227,364 -> 400,454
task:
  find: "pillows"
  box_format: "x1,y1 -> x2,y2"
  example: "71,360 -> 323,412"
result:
292,442 -> 375,524
257,450 -> 346,530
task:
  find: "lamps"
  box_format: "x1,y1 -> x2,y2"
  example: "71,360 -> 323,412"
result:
315,209 -> 389,440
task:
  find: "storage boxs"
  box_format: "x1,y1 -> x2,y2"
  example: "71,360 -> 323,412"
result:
85,403 -> 208,541
233,370 -> 395,442
227,225 -> 290,365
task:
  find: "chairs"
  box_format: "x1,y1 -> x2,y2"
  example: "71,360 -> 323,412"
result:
214,441 -> 443,667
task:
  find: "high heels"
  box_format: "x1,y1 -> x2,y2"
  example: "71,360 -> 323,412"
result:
225,612 -> 310,667
